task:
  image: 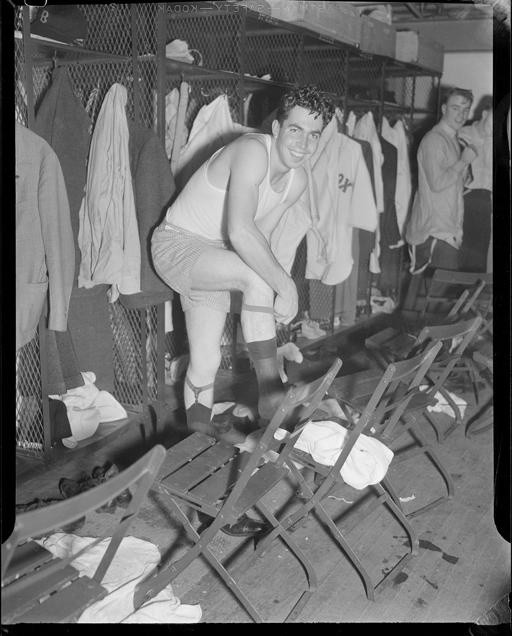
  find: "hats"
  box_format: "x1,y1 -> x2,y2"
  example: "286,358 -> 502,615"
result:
30,6 -> 88,44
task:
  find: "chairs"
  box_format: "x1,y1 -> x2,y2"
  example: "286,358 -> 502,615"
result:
321,316 -> 485,515
132,359 -> 343,624
466,352 -> 493,439
405,279 -> 485,333
0,445 -> 164,624
365,312 -> 493,440
418,270 -> 492,327
220,340 -> 444,601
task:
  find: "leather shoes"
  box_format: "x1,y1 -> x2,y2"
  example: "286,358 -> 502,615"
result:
59,472 -> 117,514
91,463 -> 133,509
207,512 -> 268,536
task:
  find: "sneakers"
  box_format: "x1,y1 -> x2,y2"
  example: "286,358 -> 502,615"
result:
15,499 -> 85,539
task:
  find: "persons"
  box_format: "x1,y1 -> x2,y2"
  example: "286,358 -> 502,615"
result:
405,86 -> 478,280
455,94 -> 493,271
149,82 -> 334,436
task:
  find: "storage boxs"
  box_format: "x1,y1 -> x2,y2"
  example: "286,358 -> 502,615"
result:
361,16 -> 396,58
397,31 -> 444,72
272,2 -> 361,47
